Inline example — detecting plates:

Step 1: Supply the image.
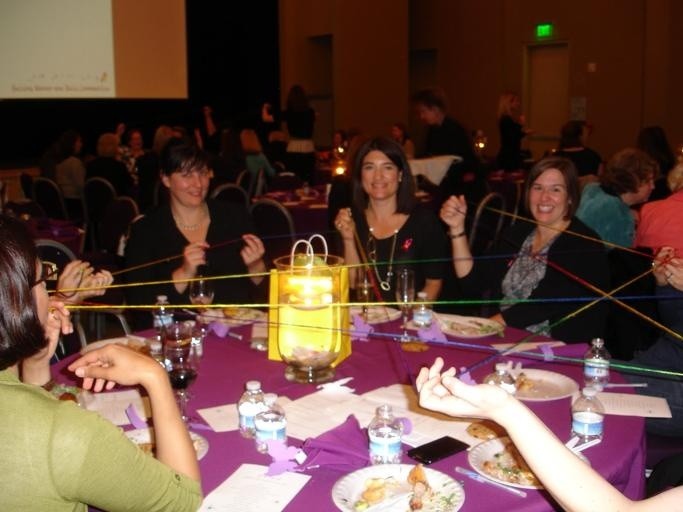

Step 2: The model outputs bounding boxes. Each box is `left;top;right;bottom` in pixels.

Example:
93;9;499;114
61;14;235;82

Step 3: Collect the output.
468;434;591;492
195;309;264;329
432;314;503;342
506;369;581;402
122;426;208;463
330;464;465;512
346;303;401;324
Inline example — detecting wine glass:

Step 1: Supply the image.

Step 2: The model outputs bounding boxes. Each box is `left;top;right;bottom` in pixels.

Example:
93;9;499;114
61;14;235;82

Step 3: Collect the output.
189;275;216;337
354;266;378;327
395;270;417;343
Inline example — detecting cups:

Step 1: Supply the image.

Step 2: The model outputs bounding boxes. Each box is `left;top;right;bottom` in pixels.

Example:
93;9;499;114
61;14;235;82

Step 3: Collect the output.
143;318;205;424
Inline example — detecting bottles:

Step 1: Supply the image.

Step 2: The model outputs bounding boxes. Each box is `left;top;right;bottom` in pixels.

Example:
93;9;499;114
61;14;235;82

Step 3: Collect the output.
239;380;265;437
153;296;173;333
569;384;603;456
583;338;612;384
412;290;433;328
484;363;516;398
367;404;401;465
253;393;286;453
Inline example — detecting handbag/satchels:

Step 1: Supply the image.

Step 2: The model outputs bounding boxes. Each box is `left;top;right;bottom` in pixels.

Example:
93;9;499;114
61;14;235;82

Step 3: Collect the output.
267;234;353;367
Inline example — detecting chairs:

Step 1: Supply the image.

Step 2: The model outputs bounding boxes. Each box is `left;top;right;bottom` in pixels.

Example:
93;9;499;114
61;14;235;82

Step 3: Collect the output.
642;432;683;489
0;127;683;368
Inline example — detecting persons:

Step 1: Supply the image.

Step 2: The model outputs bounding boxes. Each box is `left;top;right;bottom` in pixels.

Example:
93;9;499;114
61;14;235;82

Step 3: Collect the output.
0;85;683;512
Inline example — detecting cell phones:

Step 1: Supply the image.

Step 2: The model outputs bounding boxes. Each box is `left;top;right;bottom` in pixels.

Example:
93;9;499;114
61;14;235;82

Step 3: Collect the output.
406;436;469;465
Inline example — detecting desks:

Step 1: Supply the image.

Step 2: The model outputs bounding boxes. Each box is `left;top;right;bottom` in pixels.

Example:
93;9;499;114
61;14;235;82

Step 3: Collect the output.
44;307;650;512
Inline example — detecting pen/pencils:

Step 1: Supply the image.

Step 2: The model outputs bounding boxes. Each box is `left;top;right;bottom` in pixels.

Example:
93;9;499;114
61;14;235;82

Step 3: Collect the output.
226;332;243;341
455;466;528;498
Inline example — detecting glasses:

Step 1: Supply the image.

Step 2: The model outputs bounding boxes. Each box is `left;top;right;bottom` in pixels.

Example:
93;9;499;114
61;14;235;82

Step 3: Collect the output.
29;261;60;291
